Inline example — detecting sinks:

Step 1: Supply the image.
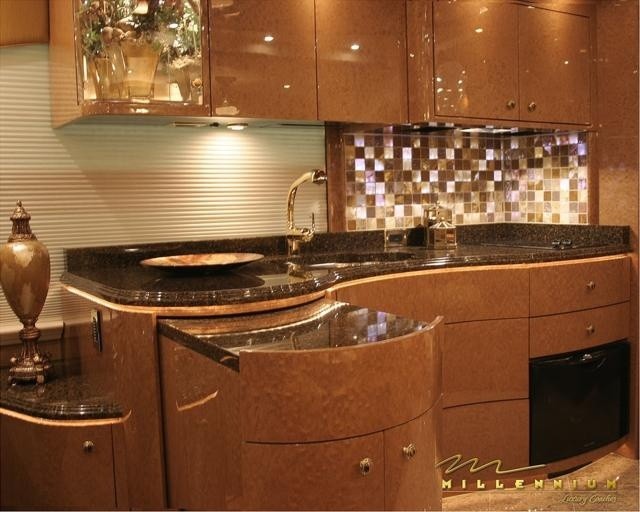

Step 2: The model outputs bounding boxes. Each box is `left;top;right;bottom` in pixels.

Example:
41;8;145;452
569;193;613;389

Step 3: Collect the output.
261;250;415;269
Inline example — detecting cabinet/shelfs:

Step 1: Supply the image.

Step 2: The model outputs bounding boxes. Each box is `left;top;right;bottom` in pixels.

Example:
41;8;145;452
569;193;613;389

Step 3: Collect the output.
435;1;597;131
2;406;128;509
210;1;410;129
160;342;445;511
527;257;630;355
338;268;529;501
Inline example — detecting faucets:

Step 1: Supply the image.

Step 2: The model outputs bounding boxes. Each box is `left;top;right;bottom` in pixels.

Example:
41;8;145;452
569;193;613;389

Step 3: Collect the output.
284;168;330;257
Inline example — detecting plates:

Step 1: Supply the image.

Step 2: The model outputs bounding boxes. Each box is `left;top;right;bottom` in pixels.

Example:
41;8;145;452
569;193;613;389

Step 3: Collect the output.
139;251;265;277
